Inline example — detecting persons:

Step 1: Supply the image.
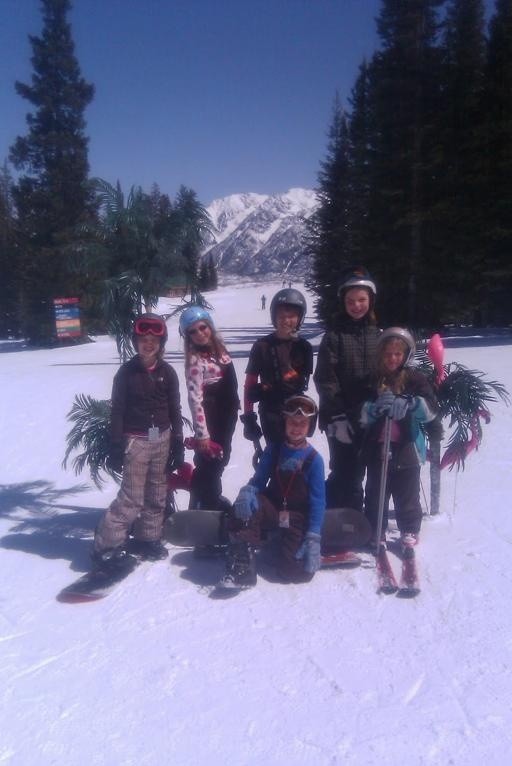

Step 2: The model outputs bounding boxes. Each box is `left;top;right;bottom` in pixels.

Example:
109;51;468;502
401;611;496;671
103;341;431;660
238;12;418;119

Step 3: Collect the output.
176;304;241;515
87;311;188;577
237;285;315;473
339;325;442;598
260;294;267;310
309;273;389;515
212;392;329;595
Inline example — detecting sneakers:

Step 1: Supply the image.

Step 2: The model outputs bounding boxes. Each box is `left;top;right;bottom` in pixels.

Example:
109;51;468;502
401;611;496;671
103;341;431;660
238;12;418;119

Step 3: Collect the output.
90;546;137;573
133;538;170;561
219;565;256;589
193;544;228;560
370;533;388;551
400;532;419;548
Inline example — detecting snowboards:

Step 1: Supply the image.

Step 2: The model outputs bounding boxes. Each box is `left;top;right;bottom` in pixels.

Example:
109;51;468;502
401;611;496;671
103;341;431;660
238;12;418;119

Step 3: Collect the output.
163;507;372;548
64;525;174;600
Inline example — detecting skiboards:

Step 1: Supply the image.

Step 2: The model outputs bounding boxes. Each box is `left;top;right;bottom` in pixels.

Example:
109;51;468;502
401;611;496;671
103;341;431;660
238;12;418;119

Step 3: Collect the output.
369;530;421;595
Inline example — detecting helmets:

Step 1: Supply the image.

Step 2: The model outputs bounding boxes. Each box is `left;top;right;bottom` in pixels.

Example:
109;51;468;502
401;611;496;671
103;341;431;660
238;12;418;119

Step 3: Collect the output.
280;394;318;438
180;306;215;338
336;276;378;296
269;288;307;332
376;327;416;367
131;313;168;353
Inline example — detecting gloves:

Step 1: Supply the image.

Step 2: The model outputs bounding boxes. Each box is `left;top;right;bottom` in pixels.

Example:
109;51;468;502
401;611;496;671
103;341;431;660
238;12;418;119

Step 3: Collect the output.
295;532;321;574
185;437;224;460
233;485;259;521
388;391;416;422
109;443;124;475
246;382;274;404
326;413;356;445
169;434;185;467
240;412;263;441
369;386;396;419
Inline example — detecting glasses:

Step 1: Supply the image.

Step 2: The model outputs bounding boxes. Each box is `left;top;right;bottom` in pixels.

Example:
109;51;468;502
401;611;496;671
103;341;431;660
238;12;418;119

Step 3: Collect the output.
340;267;372;283
135;319;166;338
186;323;208;335
282;397;317;418
